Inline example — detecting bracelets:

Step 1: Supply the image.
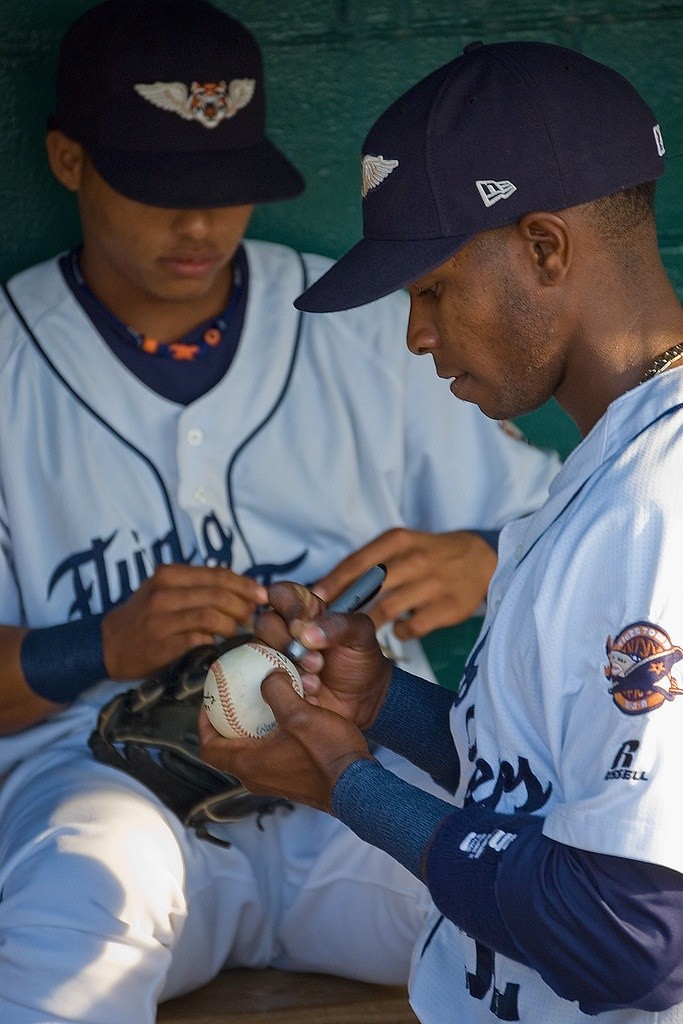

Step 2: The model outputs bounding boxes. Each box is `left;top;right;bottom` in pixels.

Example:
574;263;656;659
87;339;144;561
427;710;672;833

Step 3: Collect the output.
20;613;112;710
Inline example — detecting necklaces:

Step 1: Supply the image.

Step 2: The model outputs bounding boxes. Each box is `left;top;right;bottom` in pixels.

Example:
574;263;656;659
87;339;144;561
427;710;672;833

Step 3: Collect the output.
637;342;683;385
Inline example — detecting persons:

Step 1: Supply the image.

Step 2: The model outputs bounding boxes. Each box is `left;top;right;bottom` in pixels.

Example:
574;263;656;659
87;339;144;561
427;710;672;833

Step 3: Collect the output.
0;3;561;1024
205;40;683;1024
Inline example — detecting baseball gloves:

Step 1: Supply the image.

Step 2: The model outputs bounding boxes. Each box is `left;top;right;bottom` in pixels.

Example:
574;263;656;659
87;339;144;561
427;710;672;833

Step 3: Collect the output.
89;634;296;850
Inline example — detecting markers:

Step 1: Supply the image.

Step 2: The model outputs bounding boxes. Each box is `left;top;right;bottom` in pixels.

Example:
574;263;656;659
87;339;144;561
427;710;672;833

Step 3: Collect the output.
286;563;388;666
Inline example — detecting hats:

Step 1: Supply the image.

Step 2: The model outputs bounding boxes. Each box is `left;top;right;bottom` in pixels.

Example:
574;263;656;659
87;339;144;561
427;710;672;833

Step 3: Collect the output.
293;39;665;314
45;0;305;208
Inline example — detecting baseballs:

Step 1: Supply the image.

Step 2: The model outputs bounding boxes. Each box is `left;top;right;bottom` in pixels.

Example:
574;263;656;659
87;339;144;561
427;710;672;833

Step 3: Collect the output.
204;643;305;740
